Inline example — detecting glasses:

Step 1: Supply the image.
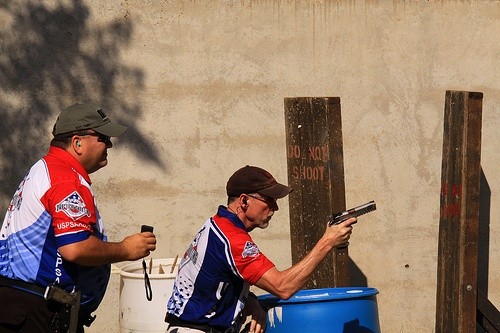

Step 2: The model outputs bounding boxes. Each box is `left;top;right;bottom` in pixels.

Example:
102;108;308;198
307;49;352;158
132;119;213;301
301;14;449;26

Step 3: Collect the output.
65;132;110;143
237;193;277;208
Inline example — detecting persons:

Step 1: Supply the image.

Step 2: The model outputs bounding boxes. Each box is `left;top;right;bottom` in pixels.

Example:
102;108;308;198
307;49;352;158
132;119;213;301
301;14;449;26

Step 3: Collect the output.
164;165;357;333
0;103;157;333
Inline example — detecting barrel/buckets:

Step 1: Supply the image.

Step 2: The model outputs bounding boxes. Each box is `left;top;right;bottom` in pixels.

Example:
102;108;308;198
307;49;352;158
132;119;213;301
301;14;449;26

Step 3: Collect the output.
119;258;183;333
257;287;381;333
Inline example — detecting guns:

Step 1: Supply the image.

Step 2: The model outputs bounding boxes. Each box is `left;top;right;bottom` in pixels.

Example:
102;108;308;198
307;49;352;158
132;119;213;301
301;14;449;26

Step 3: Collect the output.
329;200;377;248
44;284;81;333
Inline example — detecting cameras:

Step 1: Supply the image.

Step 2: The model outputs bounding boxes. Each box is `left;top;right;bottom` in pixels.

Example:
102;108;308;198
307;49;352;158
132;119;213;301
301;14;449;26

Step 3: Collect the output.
141;225;153;233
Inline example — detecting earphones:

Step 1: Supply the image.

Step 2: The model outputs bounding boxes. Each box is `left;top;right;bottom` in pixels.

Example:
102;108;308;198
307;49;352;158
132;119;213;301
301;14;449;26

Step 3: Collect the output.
243;197;247;205
77;141;80;147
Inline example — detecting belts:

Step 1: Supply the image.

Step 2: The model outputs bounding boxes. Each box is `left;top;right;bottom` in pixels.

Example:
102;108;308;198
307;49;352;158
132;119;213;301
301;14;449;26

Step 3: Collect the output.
170;318;226;333
0;275;46;298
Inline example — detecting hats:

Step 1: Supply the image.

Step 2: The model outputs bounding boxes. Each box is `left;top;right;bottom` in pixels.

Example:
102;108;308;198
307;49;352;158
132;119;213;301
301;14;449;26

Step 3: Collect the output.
52;103;128;137
227;165;294;199
